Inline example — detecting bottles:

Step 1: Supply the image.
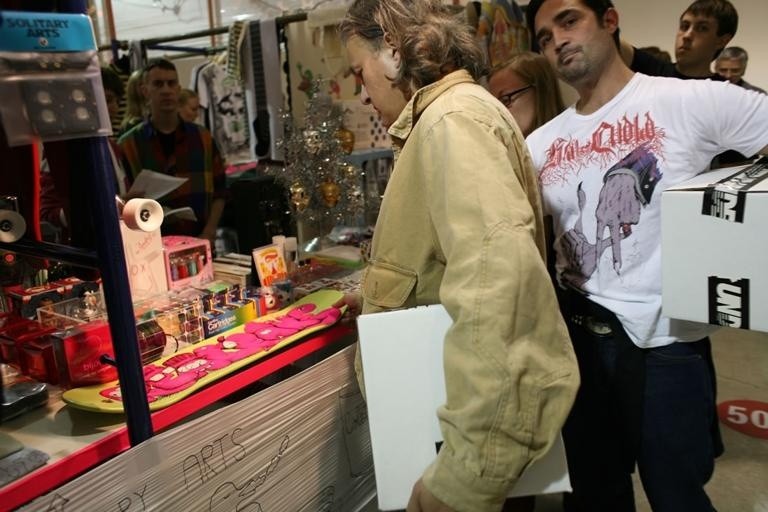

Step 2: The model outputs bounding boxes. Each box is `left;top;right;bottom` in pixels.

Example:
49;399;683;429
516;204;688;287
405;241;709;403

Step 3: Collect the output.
273;236;298;280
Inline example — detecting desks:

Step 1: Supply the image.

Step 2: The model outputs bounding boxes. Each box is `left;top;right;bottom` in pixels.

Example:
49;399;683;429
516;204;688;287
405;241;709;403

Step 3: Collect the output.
0;246;376;512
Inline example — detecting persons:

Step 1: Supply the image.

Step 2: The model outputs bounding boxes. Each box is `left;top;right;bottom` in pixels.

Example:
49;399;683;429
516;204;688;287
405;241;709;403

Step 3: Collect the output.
172;88;200;125
37;65;148;260
711;46;767;170
330;0;589;511
120;58;229;256
510;2;768;512
605;2;765;96
122;68;152;134
483;49;568;280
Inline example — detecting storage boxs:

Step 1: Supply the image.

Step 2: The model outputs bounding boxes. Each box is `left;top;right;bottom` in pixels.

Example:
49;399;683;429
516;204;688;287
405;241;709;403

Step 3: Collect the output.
659;162;768;332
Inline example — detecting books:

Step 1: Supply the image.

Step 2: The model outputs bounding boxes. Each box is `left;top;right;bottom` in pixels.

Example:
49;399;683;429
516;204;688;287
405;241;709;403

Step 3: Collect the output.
126;166;190;208
152;205;197;222
355;298;574;508
